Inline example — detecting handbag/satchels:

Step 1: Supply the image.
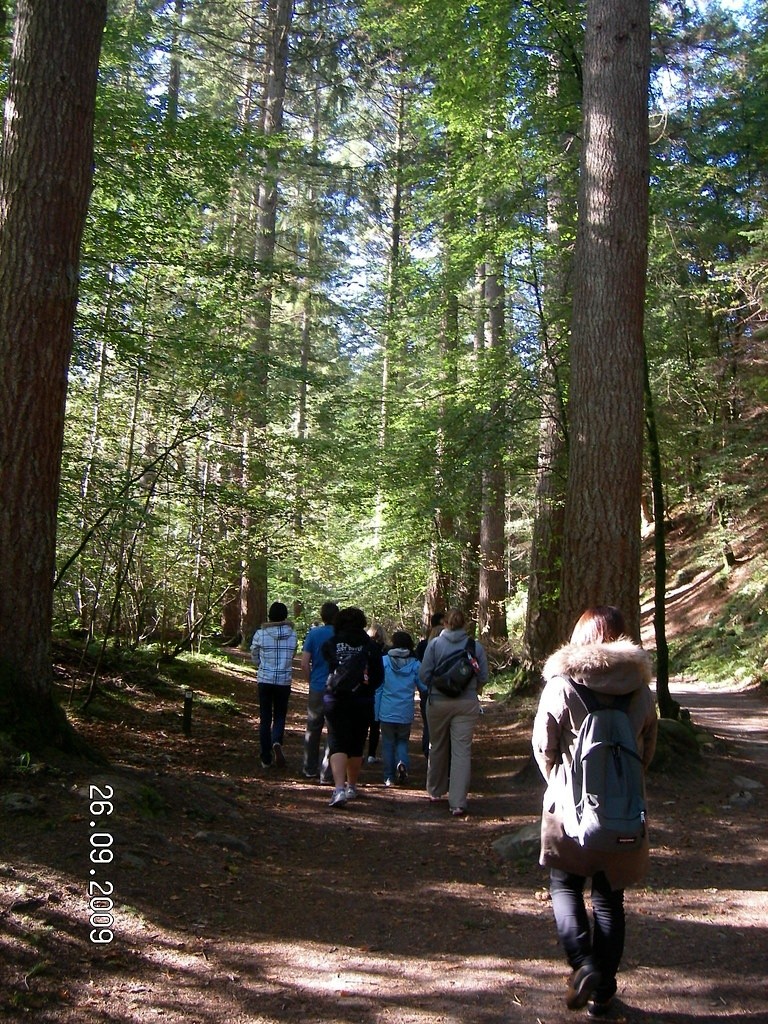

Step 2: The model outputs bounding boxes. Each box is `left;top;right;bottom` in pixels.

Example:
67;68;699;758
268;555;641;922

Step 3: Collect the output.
431;635;479;699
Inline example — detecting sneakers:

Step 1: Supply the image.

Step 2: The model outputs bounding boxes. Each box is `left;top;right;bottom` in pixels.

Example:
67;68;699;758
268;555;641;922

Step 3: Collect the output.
328;786;348;806
344;783;359;799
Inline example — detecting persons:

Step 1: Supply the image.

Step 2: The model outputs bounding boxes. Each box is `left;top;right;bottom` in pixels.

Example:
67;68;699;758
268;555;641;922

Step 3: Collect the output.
533;607;659;1024
358;624;392;761
375;630;429;787
320;606;385;807
414;615;466;780
418;609;491;815
250;602;299;768
303;602;347;784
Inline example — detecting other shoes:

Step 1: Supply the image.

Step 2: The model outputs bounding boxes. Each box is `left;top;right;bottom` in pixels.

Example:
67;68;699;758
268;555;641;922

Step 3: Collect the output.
367;756;383;763
301;766;317;778
587;978;617;1018
449;806;466;815
320;771;333;785
272;742;286;768
396;761;409;780
428;793;441;802
261;759;272;768
566;964;601;1011
384;777;396;787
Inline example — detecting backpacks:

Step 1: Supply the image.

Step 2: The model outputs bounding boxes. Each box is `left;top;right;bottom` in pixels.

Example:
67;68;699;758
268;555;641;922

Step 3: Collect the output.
330;636;372;700
548;680;648;854
